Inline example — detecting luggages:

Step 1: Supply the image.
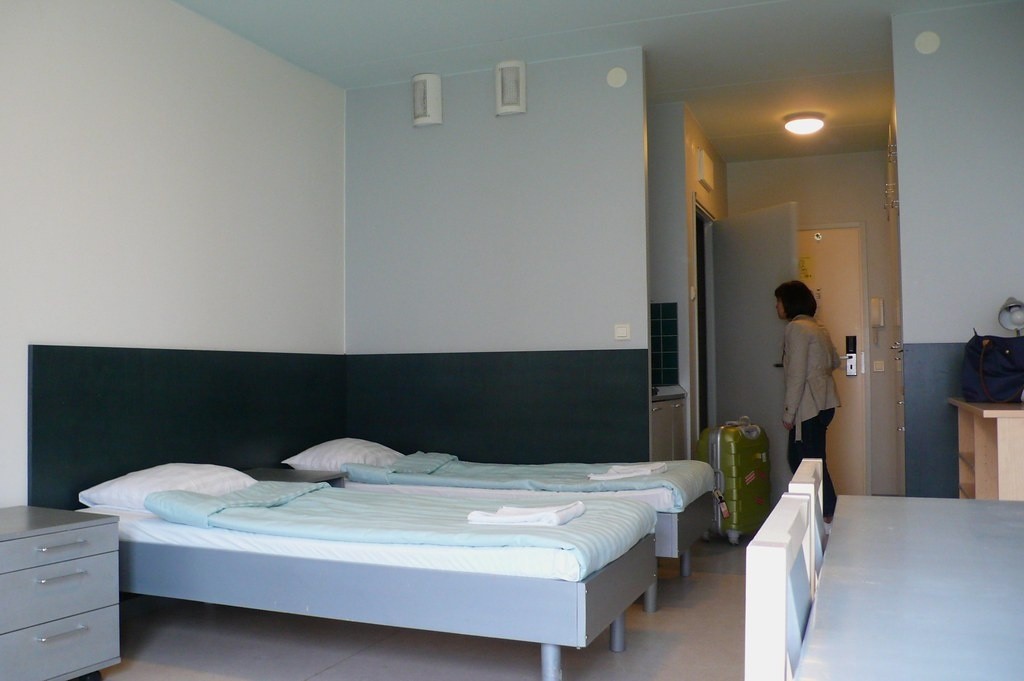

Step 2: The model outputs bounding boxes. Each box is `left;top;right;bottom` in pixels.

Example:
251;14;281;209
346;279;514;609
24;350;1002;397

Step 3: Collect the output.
696;415;770;545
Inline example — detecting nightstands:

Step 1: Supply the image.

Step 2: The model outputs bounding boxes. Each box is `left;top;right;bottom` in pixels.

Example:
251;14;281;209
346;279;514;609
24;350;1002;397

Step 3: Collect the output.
0;505;122;681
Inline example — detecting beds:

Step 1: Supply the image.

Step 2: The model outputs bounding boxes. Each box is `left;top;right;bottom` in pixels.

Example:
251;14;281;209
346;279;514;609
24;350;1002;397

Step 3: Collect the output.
75;437;717;680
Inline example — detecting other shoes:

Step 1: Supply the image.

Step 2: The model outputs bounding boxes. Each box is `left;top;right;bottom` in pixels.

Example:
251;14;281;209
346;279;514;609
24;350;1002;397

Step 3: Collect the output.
824;520;832;535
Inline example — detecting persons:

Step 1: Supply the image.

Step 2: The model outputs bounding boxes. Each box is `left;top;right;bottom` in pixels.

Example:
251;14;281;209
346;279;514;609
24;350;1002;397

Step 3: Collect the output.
775;279;843;535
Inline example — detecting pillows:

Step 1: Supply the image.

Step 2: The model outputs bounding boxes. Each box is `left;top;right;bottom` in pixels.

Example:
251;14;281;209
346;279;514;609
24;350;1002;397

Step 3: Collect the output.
280;438;404;470
77;463;256;514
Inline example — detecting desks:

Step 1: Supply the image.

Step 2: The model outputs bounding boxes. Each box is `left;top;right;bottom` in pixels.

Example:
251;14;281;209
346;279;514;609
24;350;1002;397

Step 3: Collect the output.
795;495;1024;681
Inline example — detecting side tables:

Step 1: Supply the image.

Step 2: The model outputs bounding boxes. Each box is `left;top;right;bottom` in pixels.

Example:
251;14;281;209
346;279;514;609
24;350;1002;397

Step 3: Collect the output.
247;468;348;488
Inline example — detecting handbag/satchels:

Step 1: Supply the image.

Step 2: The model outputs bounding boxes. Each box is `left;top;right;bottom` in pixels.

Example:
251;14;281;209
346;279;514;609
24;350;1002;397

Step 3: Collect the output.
963;328;1024;404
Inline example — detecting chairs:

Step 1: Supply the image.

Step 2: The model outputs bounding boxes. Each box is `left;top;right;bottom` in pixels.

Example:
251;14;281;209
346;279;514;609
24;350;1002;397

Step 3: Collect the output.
743;456;827;681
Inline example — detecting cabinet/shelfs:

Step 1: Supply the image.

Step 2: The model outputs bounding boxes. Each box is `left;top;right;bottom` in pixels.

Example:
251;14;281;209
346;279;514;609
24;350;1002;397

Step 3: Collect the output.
949;396;1024;500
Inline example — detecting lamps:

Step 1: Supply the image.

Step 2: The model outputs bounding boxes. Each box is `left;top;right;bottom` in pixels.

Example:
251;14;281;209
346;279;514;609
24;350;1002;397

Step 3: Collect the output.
998;297;1024;338
494;61;526;116
783;115;823;136
412;74;444;126
